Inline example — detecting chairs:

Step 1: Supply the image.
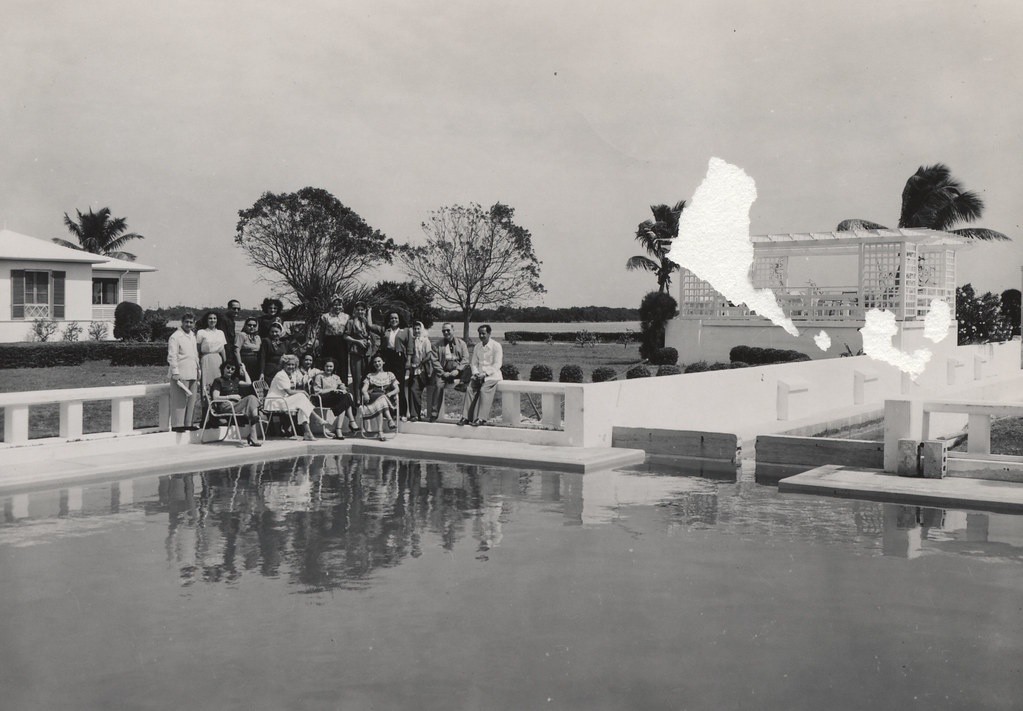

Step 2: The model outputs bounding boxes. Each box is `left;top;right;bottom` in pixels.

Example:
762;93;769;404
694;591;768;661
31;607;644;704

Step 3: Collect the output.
252;380;298;439
200;383;265;447
308;378;354;439
360;393;400;439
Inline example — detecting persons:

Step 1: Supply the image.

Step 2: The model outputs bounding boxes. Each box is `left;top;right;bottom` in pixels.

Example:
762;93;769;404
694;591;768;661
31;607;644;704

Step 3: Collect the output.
360;353;399;442
311;358;362;439
264;353;330;441
210;359;263;446
293;354;325;396
162;291;506;439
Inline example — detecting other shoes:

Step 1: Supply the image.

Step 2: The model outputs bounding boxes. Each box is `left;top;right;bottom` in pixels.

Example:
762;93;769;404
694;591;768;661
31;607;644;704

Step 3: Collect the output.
310;418;328;425
172;427;186;432
303;431;317;441
410;416;421;421
454;384;468;392
429;416;436;422
199;420;220;430
185;426;198;430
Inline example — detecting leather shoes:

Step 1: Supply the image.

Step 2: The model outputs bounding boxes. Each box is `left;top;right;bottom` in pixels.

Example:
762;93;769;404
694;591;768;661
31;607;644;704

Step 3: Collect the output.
470;418;484;425
456;418;469;426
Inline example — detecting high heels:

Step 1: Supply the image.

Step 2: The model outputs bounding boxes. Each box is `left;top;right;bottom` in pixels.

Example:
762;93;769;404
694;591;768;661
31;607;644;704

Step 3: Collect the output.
388;419;397;430
247;433;261;446
250;415;260;427
349;420;360;432
378;431;386;440
334;428;345;440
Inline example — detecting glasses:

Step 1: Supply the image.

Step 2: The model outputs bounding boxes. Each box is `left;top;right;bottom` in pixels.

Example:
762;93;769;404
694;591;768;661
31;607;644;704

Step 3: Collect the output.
230;306;241;311
442;329;450;333
226;366;235;371
247;324;257;327
